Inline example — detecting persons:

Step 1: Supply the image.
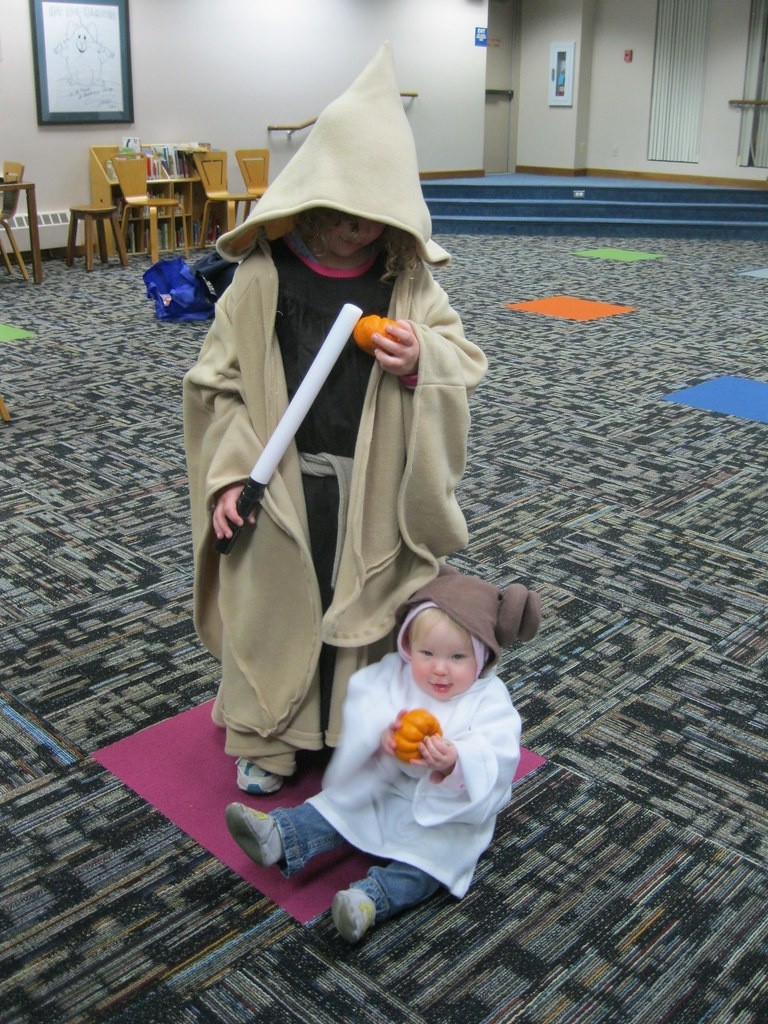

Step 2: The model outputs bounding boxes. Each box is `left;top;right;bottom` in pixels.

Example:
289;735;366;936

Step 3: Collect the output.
227;564;522;945
176;101;489;792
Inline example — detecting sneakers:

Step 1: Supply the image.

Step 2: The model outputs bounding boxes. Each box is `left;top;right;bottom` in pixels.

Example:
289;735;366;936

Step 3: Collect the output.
235;755;283;795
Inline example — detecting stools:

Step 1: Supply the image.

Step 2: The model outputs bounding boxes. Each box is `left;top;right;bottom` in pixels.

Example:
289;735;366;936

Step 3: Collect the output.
65;204;130;275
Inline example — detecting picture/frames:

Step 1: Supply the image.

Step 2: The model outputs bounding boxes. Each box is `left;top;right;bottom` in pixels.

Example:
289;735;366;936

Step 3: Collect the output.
27;1;137;126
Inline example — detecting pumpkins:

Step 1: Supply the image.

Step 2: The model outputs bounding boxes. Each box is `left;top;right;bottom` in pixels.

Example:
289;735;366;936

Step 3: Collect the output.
355;314;404;359
394;709;442;763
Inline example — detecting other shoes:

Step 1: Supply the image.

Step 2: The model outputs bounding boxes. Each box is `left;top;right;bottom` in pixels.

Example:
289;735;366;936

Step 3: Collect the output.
332;887;375;946
225;802;284;869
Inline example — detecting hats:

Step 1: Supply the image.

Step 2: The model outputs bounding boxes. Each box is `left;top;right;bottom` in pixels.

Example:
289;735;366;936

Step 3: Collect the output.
397;600;491;679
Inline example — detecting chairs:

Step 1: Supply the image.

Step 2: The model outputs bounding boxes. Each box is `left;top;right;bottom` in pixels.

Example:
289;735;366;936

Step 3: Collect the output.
110;152;192;264
0;160;30;283
192;152;255;249
237;148;275;224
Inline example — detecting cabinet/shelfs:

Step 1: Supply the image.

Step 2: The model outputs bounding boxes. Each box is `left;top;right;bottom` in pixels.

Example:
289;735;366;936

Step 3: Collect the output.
87;139;230;247
